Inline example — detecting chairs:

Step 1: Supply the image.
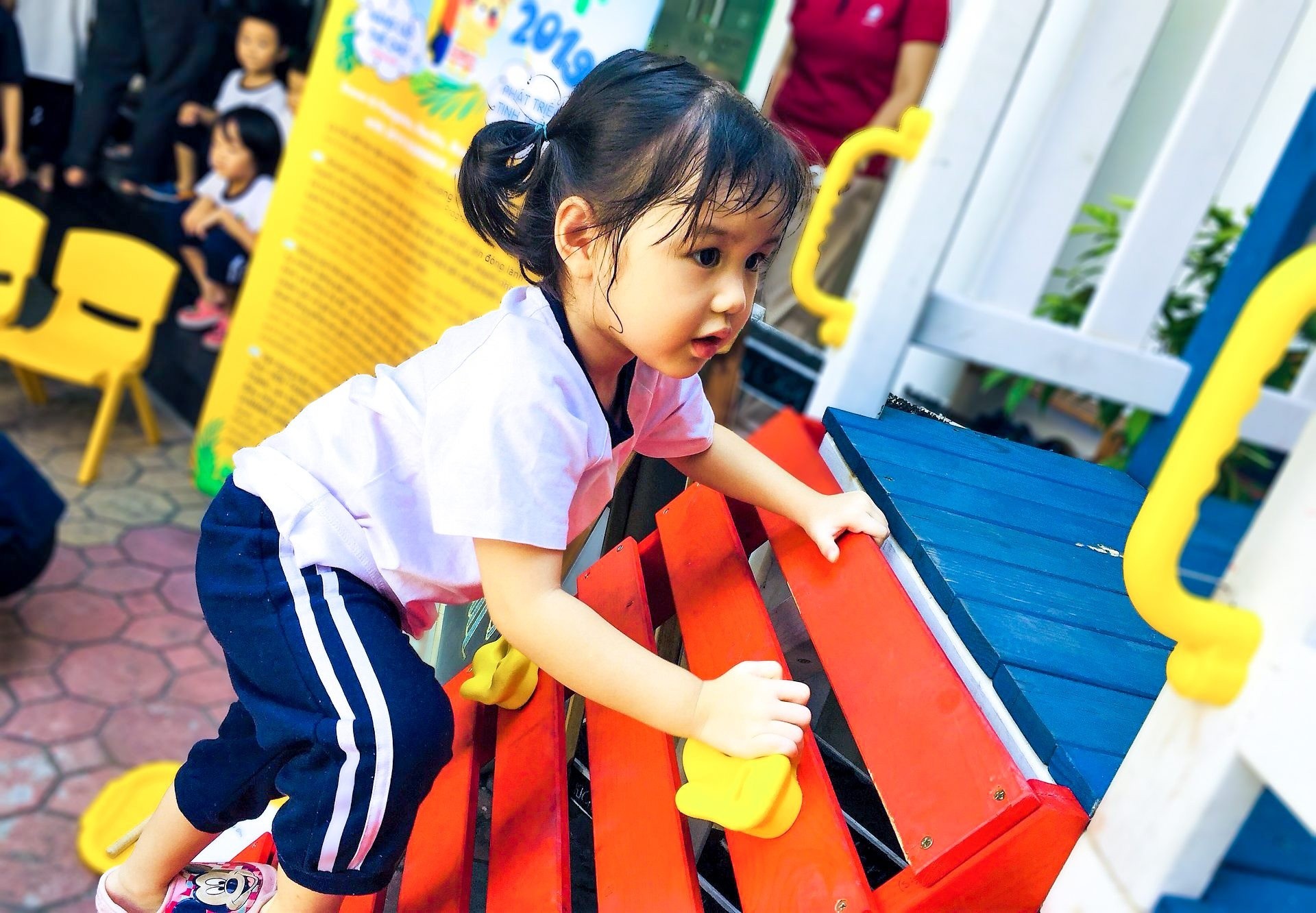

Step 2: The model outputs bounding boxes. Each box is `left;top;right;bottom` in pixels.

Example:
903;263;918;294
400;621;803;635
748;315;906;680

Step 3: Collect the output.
0;190;49;403
0;227;180;489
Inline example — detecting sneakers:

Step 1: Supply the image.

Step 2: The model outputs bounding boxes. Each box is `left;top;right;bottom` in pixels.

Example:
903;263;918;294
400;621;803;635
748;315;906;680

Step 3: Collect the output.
95;861;277;913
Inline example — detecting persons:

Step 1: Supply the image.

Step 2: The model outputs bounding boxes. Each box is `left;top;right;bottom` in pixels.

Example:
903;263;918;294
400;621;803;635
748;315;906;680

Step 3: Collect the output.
177;106;284;351
704;0;952;445
92;49;893;913
0;1;314;205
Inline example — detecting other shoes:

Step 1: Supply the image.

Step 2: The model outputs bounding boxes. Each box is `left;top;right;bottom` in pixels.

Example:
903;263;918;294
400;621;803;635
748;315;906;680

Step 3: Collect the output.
203;314;230;350
177;294;231;327
